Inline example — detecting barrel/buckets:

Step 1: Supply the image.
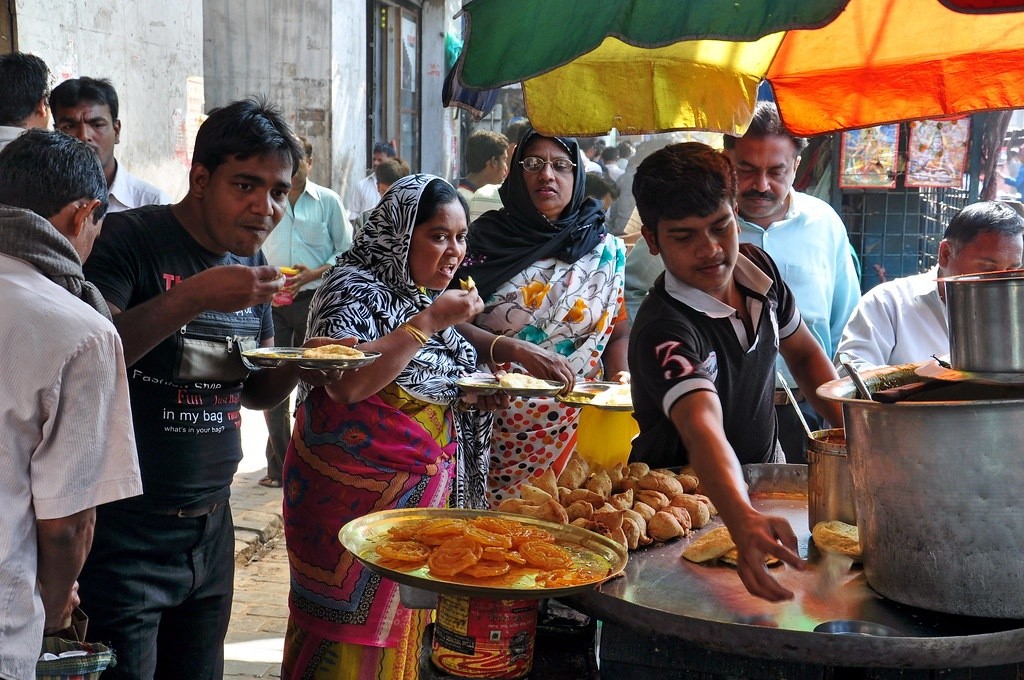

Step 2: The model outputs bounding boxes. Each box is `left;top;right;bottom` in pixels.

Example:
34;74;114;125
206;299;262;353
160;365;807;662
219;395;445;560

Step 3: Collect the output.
803;430;860;537
430;587;537;679
932;269;1024;372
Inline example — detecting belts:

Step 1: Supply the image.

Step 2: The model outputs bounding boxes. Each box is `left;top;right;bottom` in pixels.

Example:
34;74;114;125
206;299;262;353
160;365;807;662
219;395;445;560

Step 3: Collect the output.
152;504;218;518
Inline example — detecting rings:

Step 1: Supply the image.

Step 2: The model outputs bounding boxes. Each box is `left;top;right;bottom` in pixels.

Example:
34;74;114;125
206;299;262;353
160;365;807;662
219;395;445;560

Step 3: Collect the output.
488;409;497;413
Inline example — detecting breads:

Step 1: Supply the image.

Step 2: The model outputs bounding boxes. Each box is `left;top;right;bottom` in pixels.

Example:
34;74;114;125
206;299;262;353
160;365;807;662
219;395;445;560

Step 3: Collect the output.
498;451;718;549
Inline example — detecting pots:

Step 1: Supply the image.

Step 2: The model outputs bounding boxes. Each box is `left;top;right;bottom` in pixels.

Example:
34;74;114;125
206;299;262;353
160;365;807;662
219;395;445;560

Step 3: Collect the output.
815;361;1024;622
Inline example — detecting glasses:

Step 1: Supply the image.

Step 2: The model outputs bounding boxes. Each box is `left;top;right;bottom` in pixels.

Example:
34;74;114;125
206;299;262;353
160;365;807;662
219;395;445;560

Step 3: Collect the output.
519;157;576;174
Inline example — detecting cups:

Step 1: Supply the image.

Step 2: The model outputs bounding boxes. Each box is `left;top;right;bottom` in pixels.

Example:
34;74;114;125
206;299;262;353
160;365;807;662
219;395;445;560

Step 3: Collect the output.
274;267;300;305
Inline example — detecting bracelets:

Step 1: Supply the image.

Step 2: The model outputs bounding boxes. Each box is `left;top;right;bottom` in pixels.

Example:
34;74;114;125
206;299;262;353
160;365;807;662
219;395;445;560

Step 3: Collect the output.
490;335;506;367
400;323;431;348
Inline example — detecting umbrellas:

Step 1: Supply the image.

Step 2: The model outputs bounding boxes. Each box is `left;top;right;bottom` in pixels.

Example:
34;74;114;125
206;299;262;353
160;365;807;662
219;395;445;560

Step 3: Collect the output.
457;3;1018;185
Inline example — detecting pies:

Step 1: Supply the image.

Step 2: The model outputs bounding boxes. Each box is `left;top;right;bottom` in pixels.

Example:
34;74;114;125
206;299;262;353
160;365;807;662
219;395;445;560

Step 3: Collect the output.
459;275;475;292
813;520;861;556
303;344;365;360
501;372;553;389
683;526;780;564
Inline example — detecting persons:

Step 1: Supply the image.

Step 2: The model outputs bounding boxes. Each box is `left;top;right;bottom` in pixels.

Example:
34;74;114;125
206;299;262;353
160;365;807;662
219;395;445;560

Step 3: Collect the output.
446;122;631;511
356;117;727;238
995;130;1024;200
623;142;841;605
833;202;1024;382
281;174;510;680
258;135;354;489
0;128;144;680
0;52;162;213
83;92;358;680
621;101;861;463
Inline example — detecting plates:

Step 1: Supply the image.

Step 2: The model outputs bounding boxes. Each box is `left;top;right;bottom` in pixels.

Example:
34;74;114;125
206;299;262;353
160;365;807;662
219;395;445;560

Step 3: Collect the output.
555;378;633;413
338;509;629;600
240;346;382;369
455;375;564;397
813;619;905;637
